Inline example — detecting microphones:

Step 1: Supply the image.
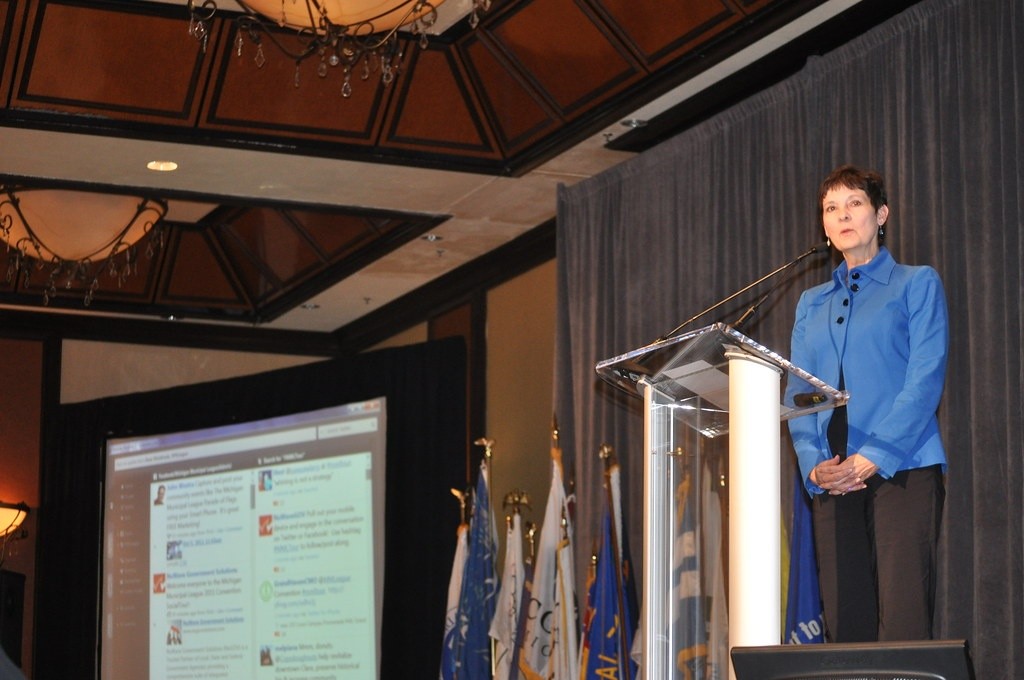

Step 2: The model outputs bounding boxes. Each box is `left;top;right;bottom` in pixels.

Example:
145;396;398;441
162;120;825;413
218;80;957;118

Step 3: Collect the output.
629;232;827;365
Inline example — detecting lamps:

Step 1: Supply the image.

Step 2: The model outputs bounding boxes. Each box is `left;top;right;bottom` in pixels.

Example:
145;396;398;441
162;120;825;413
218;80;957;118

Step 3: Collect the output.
187;0;492;96
0;501;32;564
0;185;219;303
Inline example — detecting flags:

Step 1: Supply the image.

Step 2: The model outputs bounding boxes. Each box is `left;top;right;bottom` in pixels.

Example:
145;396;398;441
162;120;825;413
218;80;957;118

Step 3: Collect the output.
435;412;824;680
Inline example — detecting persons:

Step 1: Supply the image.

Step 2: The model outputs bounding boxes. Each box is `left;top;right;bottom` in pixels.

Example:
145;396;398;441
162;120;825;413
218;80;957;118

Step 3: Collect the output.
258;471;271;491
783;164;951;641
154;486;165;503
